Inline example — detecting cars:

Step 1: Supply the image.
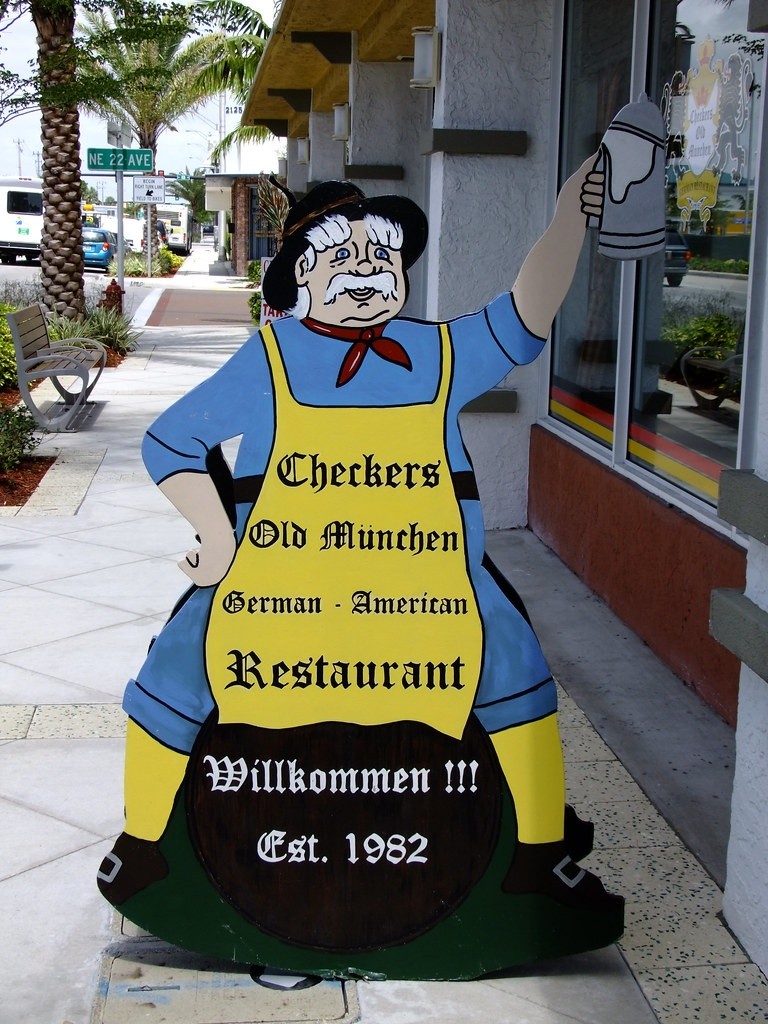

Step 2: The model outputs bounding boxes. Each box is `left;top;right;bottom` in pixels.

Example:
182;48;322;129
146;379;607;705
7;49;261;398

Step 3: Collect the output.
112;232;134;254
80;226;118;273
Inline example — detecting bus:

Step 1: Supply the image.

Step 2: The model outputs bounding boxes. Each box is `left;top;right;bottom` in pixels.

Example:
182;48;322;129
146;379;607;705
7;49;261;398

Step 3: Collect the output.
156;195;198;256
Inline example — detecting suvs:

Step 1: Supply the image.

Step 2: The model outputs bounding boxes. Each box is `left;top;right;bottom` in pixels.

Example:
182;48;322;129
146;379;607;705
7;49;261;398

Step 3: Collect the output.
666;227;692;288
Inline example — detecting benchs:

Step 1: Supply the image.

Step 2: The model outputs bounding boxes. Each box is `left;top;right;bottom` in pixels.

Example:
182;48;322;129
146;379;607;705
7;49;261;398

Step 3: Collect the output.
680;321;744;410
6;303;107;433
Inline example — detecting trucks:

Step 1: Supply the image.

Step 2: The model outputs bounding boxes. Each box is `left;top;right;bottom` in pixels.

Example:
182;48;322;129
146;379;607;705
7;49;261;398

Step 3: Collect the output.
0;177;44;264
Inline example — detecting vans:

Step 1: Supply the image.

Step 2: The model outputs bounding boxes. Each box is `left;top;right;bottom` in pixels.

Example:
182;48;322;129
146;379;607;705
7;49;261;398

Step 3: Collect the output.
156;220;167;245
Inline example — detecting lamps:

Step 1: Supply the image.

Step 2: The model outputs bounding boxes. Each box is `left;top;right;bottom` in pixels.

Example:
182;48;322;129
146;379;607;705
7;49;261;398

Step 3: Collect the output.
331;101;352;142
295;137;310;165
277;157;287;180
409;26;441;90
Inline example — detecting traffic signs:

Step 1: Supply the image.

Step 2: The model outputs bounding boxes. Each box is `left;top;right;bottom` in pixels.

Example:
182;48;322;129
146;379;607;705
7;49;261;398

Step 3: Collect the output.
133;176;165;205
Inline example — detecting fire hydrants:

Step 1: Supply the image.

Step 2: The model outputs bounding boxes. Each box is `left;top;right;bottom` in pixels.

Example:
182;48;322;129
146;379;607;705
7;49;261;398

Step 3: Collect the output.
97;278;125;317
